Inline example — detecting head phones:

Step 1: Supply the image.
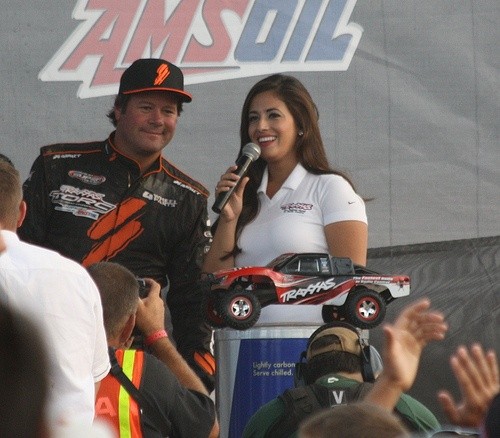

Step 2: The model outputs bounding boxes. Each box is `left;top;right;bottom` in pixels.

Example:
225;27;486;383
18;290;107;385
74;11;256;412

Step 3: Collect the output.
294;321;384;390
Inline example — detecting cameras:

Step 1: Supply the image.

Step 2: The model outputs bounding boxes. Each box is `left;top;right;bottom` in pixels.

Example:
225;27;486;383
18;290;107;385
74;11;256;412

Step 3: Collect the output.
137;279;148;299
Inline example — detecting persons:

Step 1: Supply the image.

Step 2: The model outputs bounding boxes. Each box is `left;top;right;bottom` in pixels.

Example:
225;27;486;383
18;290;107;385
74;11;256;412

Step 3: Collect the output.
242;296;500;438
201;73;368;281
0;58;218;438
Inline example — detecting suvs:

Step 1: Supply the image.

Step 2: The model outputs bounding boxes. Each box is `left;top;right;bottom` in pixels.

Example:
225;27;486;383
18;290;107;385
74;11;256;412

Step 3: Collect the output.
204;252;413;330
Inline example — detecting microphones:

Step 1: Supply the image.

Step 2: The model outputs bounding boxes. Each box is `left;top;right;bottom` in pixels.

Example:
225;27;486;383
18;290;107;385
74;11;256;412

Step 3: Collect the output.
211;143;261;214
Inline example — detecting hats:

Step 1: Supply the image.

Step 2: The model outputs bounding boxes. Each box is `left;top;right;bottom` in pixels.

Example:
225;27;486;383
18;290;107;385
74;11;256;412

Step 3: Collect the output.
306;326;366;361
118;58;193;104
